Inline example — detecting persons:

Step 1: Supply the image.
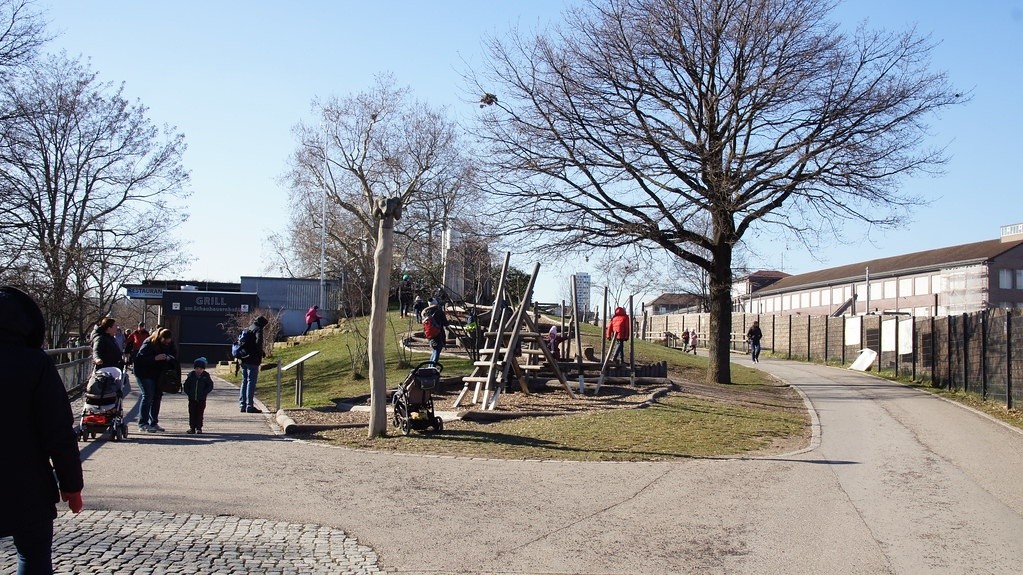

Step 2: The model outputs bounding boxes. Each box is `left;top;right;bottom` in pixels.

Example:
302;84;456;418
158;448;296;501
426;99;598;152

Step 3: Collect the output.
114;321;163;373
89;317;124;374
183;357;214;434
398;274;413;319
301;305;324;336
133;327;178;433
238;315;269;413
0;284;84;575
686;329;697;356
606;306;629;364
545;326;558;362
413;287;450;367
681;327;690;351
747;321;763;364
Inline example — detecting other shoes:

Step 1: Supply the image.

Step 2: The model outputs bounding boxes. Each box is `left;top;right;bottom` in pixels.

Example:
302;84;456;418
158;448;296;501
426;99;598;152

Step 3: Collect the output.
620;358;624;363
187;429;196;433
151;425;165;431
612;356;620;362
241;408;246;412
139;424;157;433
197;429;202;433
246;407;262;413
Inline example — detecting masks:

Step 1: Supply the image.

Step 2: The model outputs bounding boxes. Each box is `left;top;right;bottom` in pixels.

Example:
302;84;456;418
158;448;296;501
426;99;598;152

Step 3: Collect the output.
316;309;318;311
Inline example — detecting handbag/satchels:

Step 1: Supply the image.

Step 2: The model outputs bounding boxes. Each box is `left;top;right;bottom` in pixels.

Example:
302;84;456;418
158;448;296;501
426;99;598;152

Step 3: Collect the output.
159;355;182;394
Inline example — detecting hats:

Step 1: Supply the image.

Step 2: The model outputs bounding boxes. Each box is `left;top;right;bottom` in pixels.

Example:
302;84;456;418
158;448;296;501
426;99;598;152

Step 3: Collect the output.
138;321;145;327
194;357;207;369
549;326;557;343
314;305;318;309
416;296;421;301
403;275;409;279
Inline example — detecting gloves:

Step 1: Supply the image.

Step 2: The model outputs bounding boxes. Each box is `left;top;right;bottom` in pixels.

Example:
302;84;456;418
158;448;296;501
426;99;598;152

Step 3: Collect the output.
61;491;83;513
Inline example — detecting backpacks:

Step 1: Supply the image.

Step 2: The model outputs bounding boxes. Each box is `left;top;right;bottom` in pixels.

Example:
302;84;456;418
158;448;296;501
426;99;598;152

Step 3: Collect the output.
232;327;261;359
422;311;442;339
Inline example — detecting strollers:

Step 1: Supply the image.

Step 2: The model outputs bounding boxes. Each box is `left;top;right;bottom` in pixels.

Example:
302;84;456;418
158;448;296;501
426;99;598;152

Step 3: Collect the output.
79;357;128;443
391;360;444;437
